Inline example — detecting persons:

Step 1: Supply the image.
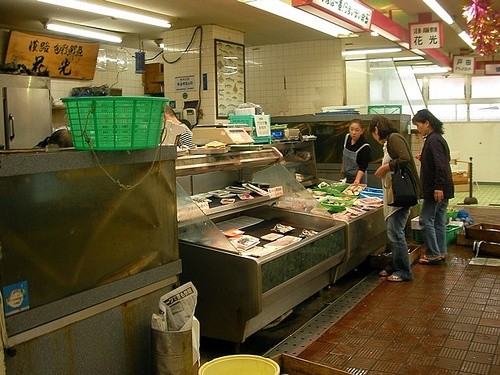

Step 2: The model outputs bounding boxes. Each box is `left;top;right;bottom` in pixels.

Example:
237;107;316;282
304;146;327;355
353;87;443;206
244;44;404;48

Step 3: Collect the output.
341;119;371;186
368;116;421;281
164;105;195;149
411;109;456;265
36;127;73;150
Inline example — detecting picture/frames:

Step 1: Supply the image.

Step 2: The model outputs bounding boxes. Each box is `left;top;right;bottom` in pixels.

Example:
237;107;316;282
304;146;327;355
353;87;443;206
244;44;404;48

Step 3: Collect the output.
214;38;246;119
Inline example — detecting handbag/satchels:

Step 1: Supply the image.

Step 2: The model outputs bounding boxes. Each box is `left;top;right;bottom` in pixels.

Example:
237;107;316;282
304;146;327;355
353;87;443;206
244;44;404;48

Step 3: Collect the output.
383;170;419;208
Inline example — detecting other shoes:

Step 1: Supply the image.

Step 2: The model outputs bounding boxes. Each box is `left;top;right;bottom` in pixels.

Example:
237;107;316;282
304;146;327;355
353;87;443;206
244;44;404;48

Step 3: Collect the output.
386;273;404;282
440;253;447;259
418;254;443;265
378;269;389;276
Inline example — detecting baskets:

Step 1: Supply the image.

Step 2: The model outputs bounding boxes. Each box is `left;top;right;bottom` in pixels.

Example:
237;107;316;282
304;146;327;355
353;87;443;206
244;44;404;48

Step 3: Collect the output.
60;95;171;152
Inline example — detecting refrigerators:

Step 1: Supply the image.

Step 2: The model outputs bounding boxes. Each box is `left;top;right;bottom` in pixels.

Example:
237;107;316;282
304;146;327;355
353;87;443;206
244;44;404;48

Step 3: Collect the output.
0;73;52;150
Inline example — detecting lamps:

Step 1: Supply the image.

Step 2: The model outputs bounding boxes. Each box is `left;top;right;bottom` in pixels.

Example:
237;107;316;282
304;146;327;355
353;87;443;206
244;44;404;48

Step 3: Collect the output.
36;0;172;29
44;18;125;44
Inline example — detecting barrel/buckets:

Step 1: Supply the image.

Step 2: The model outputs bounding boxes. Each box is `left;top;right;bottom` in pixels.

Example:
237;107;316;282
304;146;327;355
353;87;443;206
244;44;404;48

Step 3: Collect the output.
197;354;281;375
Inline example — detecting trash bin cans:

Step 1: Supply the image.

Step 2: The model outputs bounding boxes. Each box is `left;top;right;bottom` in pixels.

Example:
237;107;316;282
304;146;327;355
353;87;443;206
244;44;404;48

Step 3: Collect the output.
150;325;198;375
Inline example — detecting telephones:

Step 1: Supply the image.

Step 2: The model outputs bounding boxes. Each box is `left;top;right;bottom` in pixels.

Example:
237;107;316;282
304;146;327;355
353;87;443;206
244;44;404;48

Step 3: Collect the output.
183;108;196;125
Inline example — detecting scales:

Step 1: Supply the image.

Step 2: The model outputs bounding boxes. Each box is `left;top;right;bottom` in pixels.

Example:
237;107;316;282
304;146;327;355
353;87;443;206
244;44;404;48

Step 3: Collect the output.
191;124;255;147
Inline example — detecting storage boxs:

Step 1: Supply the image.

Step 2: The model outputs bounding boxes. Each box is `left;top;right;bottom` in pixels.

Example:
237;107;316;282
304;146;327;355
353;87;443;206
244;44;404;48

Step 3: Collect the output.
451;171;470;192
371;202;500;271
